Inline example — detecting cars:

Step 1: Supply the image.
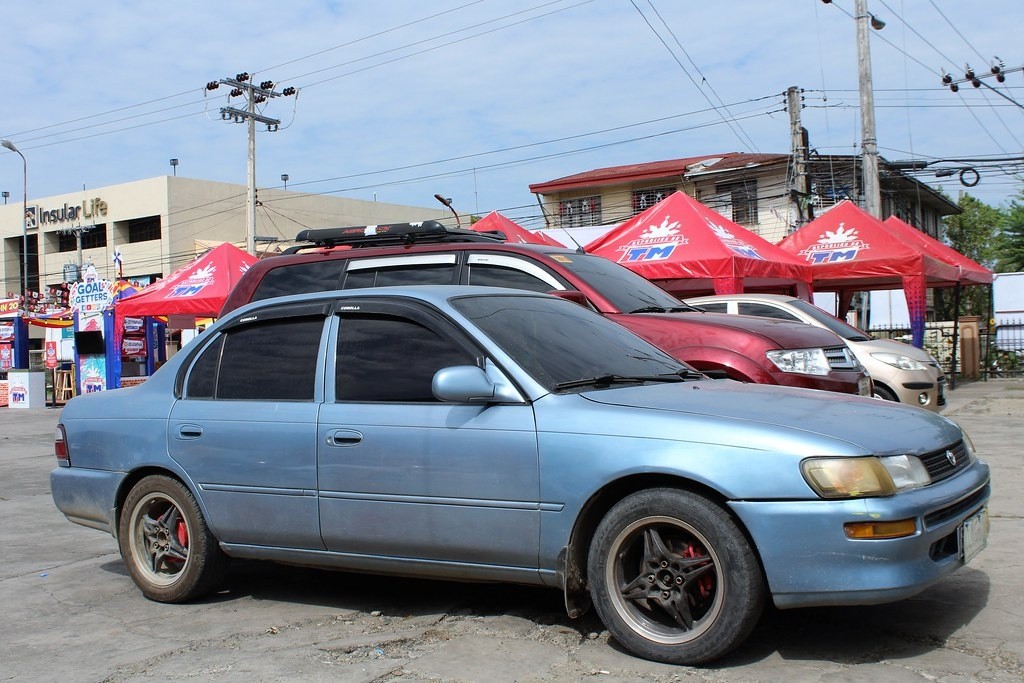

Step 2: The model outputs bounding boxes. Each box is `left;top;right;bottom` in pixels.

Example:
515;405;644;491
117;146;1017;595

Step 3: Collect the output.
674;294;949;412
53;281;994;672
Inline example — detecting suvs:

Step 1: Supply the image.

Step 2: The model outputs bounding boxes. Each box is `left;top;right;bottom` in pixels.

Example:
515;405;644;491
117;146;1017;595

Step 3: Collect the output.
214;224;878;402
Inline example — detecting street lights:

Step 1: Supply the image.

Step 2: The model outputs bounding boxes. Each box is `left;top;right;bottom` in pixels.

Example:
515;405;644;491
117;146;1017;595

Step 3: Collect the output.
0;139;30;317
